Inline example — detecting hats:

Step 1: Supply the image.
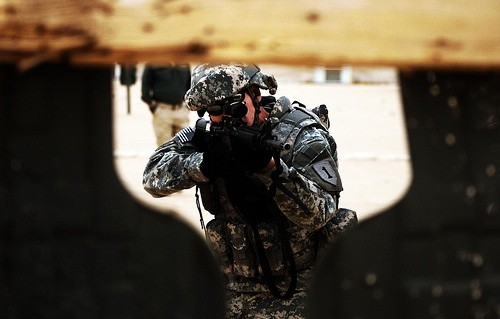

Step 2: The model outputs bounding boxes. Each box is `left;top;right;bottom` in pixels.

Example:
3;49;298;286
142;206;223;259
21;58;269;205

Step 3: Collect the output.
184;62;260;111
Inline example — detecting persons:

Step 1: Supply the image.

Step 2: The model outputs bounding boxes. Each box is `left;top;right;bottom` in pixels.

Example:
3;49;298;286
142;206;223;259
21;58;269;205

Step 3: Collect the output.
140;63;191;148
143;62;358;319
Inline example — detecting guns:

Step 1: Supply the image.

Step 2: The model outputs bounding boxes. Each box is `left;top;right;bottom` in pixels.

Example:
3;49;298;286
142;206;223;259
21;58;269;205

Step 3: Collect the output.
191;92;290;171
